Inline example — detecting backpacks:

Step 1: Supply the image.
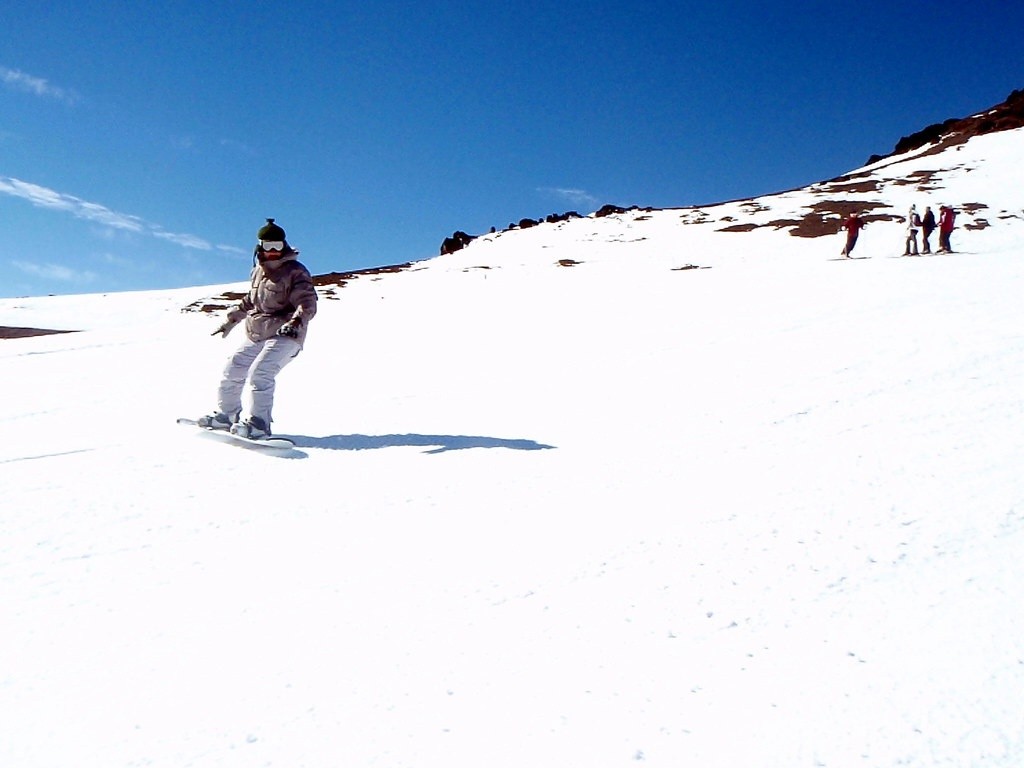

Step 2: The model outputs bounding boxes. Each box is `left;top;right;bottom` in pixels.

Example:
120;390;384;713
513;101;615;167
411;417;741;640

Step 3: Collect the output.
912;213;921;226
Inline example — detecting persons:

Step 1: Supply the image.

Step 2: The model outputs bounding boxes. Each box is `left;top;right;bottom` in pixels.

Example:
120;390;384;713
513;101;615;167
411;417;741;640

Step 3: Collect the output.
920;206;937;254
902;205;922;257
841;211;864;257
199;218;317;439
936;205;954;254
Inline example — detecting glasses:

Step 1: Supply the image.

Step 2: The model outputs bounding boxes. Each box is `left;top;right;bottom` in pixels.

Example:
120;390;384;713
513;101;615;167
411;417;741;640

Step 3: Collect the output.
262;240;284;251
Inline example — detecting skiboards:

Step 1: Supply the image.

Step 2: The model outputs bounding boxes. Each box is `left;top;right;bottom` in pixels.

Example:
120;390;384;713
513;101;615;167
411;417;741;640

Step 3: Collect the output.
888;251;982;258
825;256;873;261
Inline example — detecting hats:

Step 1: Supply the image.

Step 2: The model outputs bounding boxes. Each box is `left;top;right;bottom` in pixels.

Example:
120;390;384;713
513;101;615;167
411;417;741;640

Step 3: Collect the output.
909;204;915;211
257;219;286;252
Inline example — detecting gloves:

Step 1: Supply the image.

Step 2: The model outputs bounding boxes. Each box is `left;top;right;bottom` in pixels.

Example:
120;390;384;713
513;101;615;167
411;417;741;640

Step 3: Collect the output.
276;319;302;339
211;320;238;338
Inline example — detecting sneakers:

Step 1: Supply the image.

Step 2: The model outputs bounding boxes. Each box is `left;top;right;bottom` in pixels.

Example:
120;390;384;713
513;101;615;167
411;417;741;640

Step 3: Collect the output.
230;415;271;439
198;411;241;430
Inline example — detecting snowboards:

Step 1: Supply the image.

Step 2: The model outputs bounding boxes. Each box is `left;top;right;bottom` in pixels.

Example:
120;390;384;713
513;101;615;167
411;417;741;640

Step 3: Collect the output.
176;418;296;450
